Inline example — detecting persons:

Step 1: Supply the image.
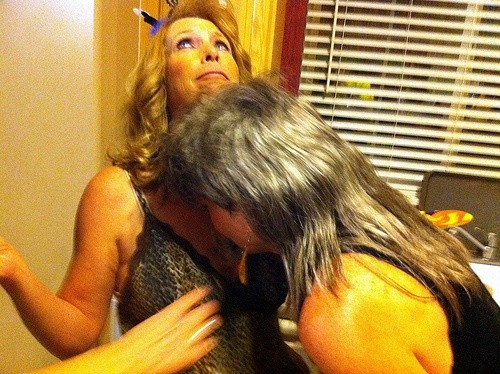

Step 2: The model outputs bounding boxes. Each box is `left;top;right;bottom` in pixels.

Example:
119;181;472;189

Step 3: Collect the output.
157;79;500;374
0;0;313;374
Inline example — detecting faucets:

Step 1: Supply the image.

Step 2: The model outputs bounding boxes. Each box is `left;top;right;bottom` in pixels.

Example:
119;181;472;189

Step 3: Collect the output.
450;222;498;260
